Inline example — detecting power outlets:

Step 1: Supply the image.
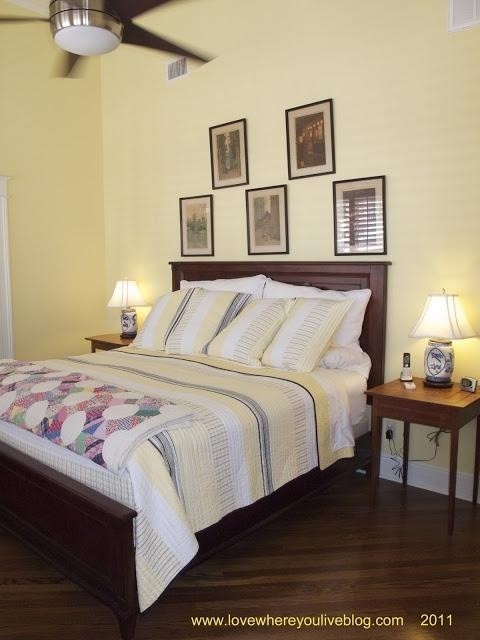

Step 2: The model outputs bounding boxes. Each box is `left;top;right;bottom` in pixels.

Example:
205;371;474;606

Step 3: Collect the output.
382;420;395;439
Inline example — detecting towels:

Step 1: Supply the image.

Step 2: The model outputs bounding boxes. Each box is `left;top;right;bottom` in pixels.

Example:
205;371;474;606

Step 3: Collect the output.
1;359;194;476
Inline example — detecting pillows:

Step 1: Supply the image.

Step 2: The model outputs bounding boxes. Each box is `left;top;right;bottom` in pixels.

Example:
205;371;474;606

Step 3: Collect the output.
207;297;302;369
128;288;201;351
263;278;372;380
261;298;355;373
180;274;267;299
163;288;255;355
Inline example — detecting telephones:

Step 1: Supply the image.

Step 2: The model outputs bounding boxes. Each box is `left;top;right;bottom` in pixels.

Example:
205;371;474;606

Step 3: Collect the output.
400;352;412;382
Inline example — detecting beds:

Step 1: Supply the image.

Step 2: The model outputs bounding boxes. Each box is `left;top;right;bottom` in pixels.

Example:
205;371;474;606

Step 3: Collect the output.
0;262;394;640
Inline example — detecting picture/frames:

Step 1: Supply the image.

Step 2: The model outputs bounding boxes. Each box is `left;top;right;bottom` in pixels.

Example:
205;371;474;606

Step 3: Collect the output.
333;176;387;255
209;118;249;189
179;195;214;256
245;185;289;255
285;99;336;179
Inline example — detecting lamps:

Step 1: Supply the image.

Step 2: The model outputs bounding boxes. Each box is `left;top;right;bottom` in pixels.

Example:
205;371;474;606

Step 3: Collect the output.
107;280;149;339
409;288;479;388
49;10;124;56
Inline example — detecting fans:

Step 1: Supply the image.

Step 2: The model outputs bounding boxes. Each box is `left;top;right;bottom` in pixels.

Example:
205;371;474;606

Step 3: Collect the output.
0;1;207;77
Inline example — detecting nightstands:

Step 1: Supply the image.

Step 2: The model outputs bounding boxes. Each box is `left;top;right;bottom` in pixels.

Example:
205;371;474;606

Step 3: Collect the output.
85;333;134;354
364;378;480;534
